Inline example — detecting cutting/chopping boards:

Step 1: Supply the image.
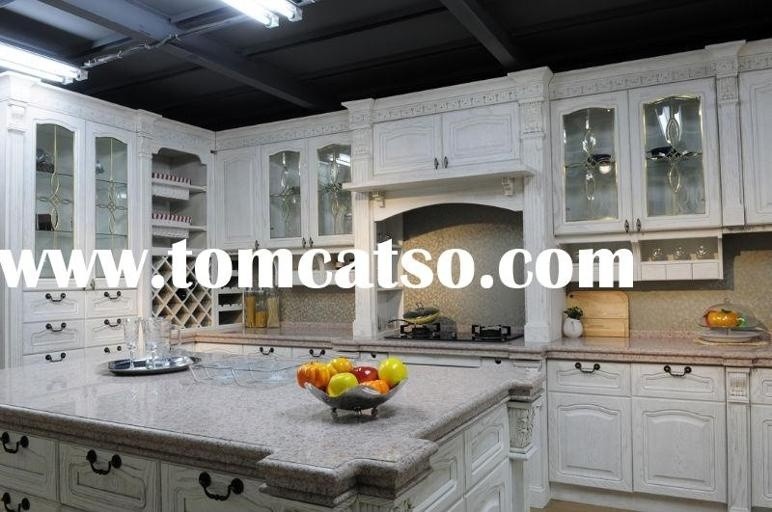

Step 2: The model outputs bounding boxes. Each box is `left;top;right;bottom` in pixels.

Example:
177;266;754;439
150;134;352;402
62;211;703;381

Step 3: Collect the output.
566;291;630;339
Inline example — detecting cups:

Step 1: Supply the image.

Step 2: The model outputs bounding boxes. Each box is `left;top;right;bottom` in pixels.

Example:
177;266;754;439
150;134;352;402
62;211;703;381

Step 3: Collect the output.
140;316;180;369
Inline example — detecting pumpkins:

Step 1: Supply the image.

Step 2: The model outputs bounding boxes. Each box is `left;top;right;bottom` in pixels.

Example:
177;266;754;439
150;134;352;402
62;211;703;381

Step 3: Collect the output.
296;358;353;389
705;308;744;327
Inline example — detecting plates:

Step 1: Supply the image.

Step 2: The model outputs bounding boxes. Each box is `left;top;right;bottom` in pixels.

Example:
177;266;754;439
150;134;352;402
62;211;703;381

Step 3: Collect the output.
696;330;761;344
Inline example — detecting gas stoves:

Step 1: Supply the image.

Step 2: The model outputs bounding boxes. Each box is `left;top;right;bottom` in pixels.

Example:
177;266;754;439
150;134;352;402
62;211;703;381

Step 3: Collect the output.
384;323;524;343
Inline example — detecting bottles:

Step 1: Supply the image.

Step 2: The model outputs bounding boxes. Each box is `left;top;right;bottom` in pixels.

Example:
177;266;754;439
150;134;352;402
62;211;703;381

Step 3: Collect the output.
255;286;266;328
267;287;280;328
245;285;255;328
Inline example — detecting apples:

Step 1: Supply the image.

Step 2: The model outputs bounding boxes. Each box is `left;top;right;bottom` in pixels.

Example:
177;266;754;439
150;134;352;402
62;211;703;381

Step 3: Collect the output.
329;358;408;397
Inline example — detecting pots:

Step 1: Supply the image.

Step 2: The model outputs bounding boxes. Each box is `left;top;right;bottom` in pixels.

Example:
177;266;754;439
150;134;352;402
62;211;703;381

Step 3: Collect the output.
387;302;440;325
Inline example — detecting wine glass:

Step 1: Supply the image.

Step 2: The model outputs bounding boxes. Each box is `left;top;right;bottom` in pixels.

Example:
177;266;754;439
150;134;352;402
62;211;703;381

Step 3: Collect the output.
120;314;142;368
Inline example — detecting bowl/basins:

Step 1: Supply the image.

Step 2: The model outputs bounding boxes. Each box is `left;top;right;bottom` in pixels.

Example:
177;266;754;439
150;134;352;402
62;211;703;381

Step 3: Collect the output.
650;146;676;156
586;154;611;163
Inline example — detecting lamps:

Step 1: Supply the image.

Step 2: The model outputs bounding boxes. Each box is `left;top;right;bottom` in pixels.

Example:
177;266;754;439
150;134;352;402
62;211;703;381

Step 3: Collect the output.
0;42;88;85
224;0;303;28
